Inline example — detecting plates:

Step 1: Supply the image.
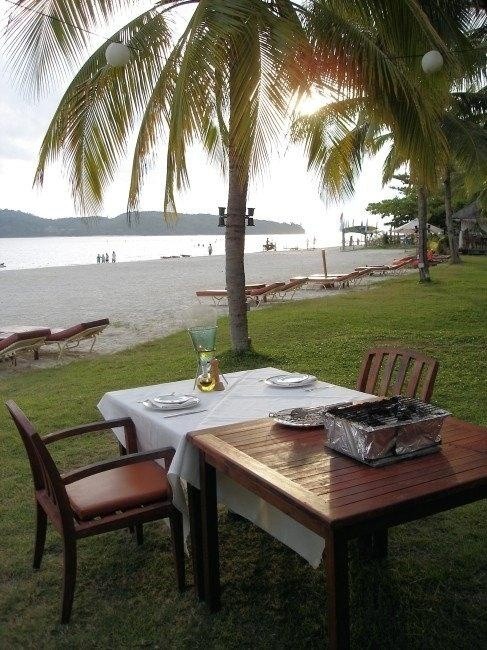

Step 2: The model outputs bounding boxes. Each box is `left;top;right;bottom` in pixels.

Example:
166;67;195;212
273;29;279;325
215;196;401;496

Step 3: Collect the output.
154;394;190;404
273;408;328;427
271;373;309;383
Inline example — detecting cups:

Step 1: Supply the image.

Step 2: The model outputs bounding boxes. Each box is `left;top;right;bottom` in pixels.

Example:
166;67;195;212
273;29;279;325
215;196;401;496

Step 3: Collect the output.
188;326;218;392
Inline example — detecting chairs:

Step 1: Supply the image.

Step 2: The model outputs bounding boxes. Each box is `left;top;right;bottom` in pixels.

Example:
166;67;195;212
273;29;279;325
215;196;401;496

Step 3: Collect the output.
0;329;51;366
1;319;110;359
5;400;186;625
357;348;440;404
195;254;451;310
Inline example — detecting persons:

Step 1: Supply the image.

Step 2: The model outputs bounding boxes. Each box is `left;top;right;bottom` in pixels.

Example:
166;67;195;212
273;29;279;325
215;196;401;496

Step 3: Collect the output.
104;253;109;262
111;251;116;262
207;244;212;255
95;253;100;263
100;254;105;263
